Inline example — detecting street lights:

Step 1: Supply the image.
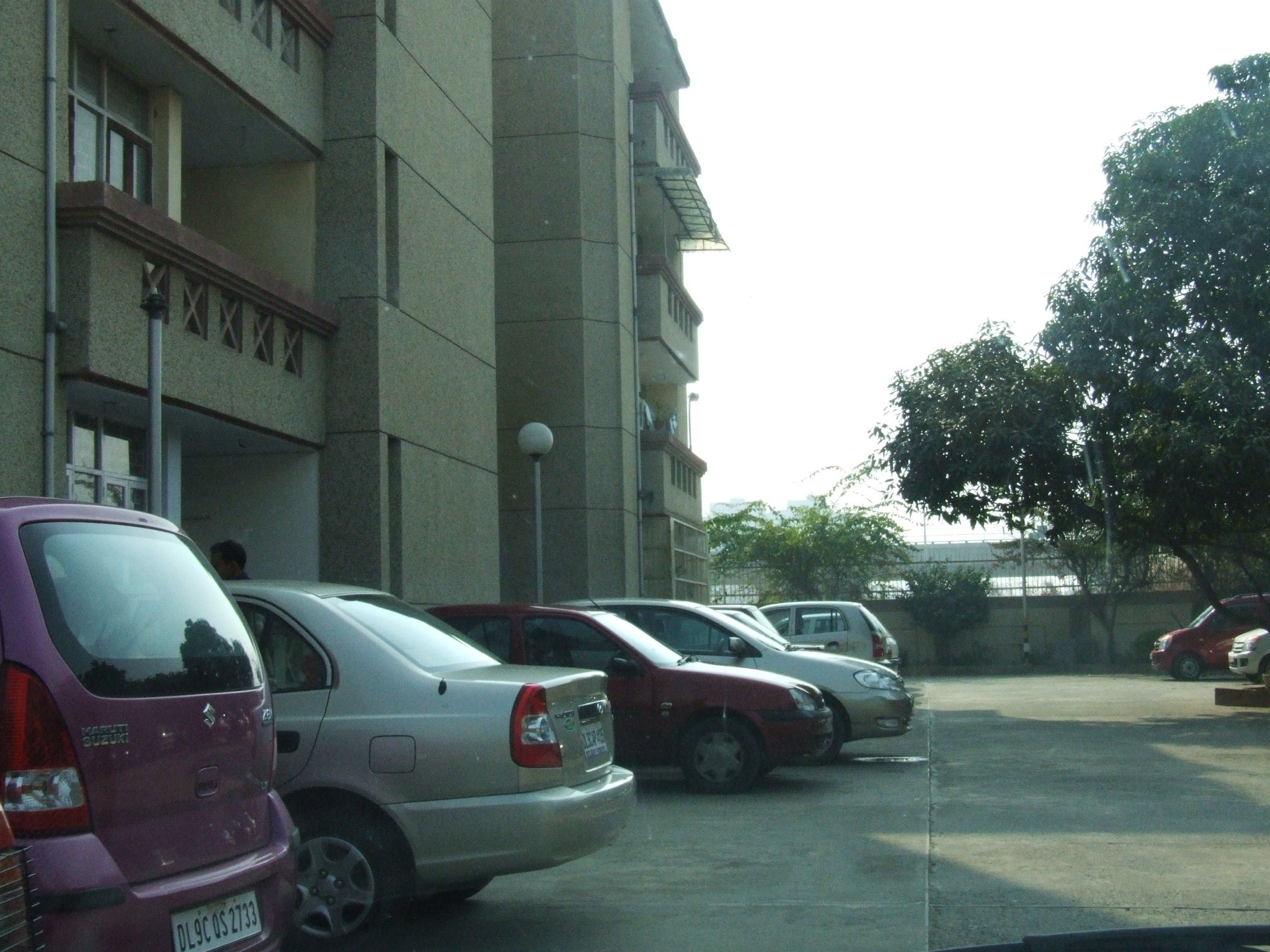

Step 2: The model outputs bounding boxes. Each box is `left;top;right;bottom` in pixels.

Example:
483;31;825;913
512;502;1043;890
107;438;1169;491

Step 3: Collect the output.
518;419;556;601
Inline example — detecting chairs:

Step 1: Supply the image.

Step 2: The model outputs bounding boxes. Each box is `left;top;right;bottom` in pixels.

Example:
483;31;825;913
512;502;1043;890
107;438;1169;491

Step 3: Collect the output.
641;616;664;640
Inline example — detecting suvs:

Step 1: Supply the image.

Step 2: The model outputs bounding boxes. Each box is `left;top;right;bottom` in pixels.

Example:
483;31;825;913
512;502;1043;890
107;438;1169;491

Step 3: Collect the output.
1148;595;1270;681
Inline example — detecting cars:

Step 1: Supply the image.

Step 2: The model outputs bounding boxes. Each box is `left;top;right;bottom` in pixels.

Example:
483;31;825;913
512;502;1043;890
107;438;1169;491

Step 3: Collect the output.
224;584;632;952
428;601;912;793
0;494;300;952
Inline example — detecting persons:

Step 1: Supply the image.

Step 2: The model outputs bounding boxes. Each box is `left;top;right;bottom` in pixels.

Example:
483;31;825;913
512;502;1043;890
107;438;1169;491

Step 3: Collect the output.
210;539;250;580
